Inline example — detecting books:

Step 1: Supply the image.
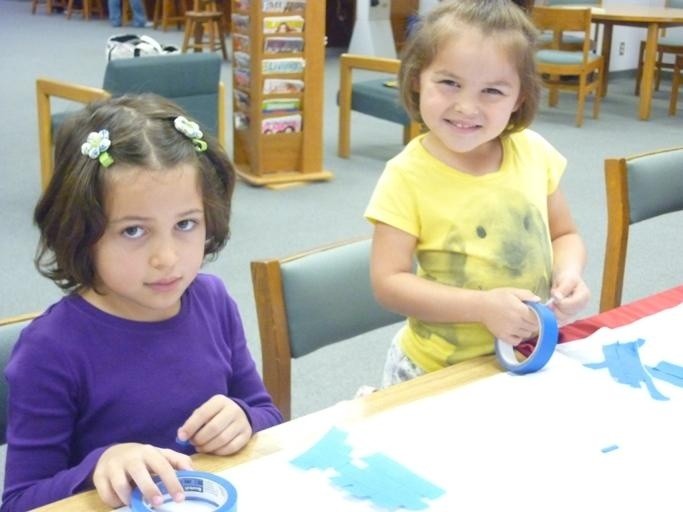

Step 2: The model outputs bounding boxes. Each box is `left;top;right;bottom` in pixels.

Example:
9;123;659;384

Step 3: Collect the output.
230;1;305;136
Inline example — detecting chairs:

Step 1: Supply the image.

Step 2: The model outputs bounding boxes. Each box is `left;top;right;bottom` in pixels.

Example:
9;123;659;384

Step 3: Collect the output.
39;55;227;188
600;149;683;313
339;53;424;159
527;2;681;128
251;237;418;422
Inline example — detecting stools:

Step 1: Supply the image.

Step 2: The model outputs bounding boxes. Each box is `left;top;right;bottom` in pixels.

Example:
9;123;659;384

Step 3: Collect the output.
181;11;228;60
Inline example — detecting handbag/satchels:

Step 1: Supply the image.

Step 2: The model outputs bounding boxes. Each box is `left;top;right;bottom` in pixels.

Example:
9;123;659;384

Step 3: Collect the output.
106;33;181;62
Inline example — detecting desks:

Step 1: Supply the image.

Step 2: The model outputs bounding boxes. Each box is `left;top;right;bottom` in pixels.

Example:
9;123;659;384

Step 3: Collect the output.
22;289;682;512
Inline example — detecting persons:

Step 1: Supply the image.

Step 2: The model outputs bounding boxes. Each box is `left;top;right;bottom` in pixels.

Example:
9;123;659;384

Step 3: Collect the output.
0;92;282;510
346;1;396;85
362;1;590;380
107;1;155;30
